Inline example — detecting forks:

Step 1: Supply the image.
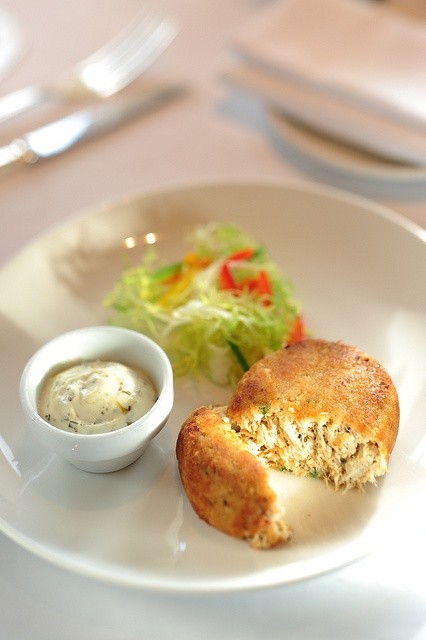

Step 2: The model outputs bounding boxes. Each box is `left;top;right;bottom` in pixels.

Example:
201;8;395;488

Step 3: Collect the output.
0;12;183;125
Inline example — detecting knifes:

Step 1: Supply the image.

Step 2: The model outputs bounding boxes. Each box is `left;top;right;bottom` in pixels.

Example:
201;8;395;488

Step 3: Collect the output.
0;78;183;169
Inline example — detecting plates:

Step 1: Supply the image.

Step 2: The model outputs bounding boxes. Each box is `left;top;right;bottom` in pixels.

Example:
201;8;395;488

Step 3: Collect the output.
267;101;425;201
1;173;426;596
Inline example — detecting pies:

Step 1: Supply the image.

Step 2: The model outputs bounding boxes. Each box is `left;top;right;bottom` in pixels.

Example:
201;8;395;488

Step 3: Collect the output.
176;339;400;550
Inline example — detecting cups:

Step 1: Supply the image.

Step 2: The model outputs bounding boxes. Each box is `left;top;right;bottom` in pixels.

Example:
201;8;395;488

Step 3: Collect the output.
17;324;176;476
